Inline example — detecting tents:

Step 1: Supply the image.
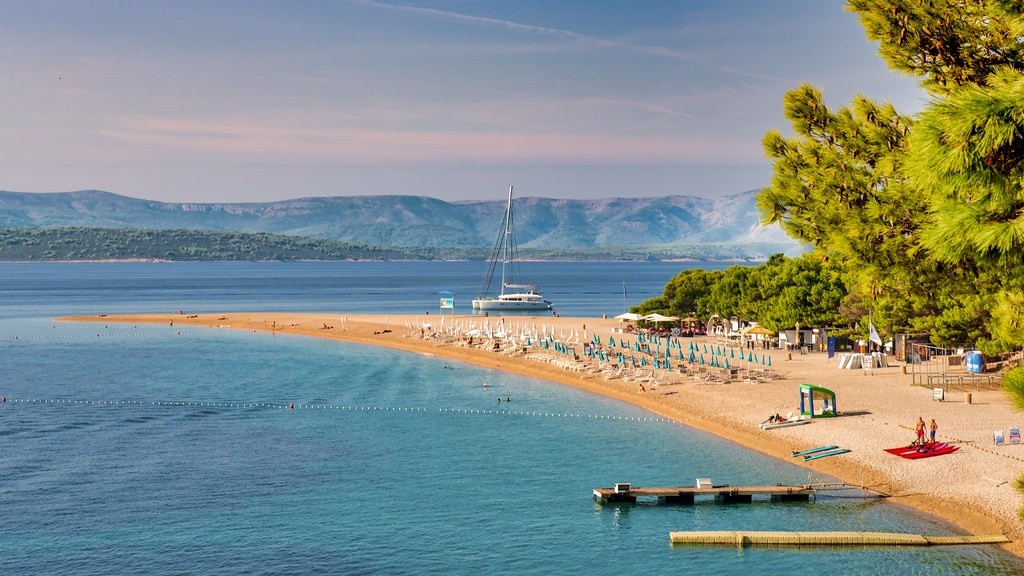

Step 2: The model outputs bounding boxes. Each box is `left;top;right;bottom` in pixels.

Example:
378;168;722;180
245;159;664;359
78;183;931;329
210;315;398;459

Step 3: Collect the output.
745;324;775;350
614;312;679;336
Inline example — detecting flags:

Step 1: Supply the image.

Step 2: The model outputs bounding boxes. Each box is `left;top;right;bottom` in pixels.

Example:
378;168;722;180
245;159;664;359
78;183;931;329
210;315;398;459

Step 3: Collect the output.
870;320;882;346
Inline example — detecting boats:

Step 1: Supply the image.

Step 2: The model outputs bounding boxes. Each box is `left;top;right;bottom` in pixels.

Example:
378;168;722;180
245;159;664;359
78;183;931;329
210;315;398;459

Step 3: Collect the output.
471;185;550;311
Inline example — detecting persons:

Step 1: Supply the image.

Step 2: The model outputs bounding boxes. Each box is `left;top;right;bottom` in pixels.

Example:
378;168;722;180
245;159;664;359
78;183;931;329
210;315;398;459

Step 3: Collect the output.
930;418;938;443
914;416;927;445
